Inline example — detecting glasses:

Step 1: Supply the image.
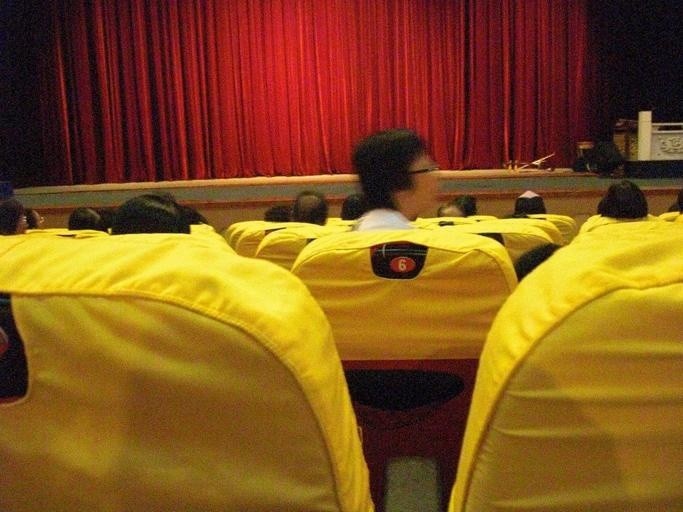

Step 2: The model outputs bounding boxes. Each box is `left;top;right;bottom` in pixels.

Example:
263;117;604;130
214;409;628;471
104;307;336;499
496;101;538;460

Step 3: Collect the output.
407;165;441;178
21;215;45;225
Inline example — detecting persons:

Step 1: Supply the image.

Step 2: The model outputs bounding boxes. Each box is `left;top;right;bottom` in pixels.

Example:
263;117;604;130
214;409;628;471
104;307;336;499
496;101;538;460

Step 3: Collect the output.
353;128;444;231
1;193;209;235
437;180;683;219
264;190;371;226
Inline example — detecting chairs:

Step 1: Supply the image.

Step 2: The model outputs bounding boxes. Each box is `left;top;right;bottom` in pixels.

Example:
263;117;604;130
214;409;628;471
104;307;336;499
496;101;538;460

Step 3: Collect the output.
0;211;683;512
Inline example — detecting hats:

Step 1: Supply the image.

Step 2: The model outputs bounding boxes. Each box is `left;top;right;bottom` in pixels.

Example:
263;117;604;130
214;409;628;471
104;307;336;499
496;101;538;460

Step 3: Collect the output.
514;191;545;217
